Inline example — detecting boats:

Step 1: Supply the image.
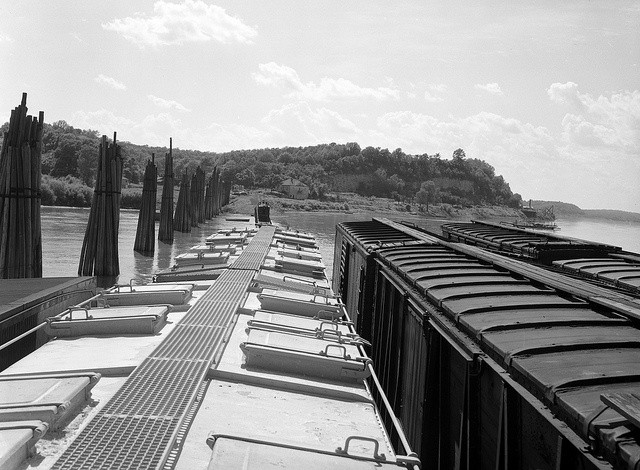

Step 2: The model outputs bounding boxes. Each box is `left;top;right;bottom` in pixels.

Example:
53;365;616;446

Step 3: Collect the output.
331;216;638;469
438;219;640;322
501;220;558;230
1;226;420;469
